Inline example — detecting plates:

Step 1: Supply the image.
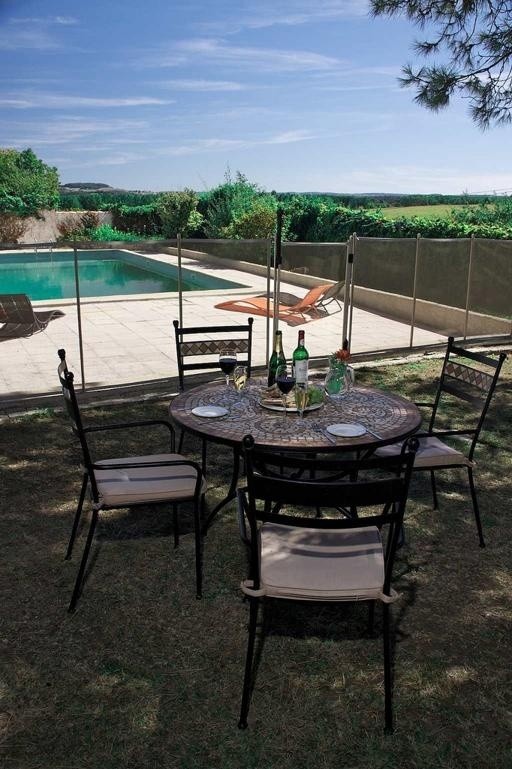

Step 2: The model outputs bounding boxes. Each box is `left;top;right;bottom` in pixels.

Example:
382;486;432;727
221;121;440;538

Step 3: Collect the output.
193;406;227;418
327;424;365;437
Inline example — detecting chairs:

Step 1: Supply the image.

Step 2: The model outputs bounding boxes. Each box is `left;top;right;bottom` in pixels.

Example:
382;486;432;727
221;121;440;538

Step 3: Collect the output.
214;280;348;327
1;293;64;338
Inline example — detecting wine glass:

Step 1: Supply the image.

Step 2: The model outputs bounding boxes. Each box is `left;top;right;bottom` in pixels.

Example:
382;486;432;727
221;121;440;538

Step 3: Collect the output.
219;349;237;389
275;364;294;418
294;382;306;423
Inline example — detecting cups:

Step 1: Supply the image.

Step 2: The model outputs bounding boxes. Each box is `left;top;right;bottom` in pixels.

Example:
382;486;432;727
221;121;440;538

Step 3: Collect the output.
232;366;248;389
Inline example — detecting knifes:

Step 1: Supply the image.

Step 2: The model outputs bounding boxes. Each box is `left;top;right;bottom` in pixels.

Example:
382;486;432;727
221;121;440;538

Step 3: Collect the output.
350;421;382;439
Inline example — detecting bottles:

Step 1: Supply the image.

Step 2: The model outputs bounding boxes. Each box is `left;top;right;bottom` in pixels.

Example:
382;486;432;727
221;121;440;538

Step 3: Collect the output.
268;330;287;388
292;330;309;388
